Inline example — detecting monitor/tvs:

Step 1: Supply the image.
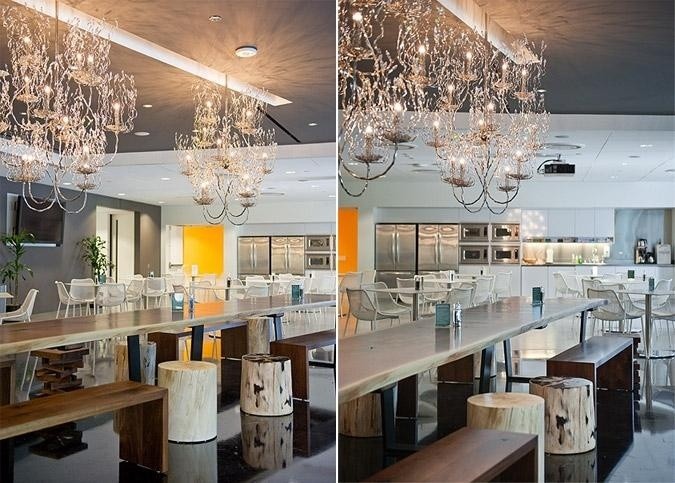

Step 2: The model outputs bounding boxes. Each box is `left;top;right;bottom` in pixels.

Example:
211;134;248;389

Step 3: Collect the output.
14;196;65;244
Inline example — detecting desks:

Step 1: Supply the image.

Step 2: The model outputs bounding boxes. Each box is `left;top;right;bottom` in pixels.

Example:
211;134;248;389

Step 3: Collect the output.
63;282;99;317
338;296;611;469
1;292;336;382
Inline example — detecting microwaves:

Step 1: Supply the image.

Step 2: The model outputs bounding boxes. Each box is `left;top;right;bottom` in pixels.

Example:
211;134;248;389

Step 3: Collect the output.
459;223;521;265
304;235;336;271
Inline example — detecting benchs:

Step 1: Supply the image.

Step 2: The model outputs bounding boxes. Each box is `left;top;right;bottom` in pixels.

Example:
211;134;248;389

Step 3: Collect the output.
546;336;635;406
1;381;170;482
269;330;336;457
147;319;249;400
594;390;635;483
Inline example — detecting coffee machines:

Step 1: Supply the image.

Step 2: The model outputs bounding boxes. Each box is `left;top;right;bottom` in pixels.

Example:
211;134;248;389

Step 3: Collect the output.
634;239;647;264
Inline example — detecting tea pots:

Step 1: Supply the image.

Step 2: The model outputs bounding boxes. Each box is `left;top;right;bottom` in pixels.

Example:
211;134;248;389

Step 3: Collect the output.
646;252;656;264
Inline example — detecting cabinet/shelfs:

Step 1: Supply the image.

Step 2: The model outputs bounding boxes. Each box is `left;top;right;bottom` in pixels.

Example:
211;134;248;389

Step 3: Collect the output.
459;207;521;224
521;209;576;239
575;207;614;239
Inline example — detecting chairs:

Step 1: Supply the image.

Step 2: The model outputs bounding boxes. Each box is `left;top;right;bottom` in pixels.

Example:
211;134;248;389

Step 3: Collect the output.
414;274;448;314
444;288;474;310
552;272;583;299
107;277;116;284
566;275;581;291
603;285;646;332
277;273;293;280
651;286;675;348
453;274;475;280
343;288;400;338
69;278;95;316
459;282;477;308
246;275;269;287
0;289;39;326
338;272;364;318
626;278;650;304
200;275;215;288
142;277;166;310
602;273;622;282
98;283;125;315
365;282;412;329
124;279;145;311
190;281;225;302
474;278;493;307
396;278;427;319
55;281;93;319
283;277;309;302
268;275;279;295
636;278;672;339
232;283;277;302
586;289;649;359
167;275;189;296
362;270;376;285
582;279;605;339
230;279;245;301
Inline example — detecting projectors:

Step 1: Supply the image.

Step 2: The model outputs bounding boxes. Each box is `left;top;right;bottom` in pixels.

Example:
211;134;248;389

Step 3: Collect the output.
543;164;576;176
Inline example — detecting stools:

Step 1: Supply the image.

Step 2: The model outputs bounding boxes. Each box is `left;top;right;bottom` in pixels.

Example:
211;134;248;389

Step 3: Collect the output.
166;438;220;483
156;361;218;444
544;449;597;482
114;341;157;387
465;392;547;483
241;411;294;471
529;376;597;455
111;411;122;435
338;392;383;438
240;353;295;417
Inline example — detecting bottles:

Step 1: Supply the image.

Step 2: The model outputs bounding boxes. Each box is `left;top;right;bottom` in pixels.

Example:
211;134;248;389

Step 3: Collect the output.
188;295;194;312
540;286;546;303
454;327;461;348
299;289;303;302
453;303;462;326
415;277;420;290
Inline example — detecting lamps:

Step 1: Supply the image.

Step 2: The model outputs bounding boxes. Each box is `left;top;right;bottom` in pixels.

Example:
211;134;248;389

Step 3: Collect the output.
338;0;426;197
1;2;138;212
426;1;547;213
172;75;277;226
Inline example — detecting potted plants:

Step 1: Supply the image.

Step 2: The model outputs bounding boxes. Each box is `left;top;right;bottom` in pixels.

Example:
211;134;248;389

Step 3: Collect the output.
1;227;35;323
74;235;116;309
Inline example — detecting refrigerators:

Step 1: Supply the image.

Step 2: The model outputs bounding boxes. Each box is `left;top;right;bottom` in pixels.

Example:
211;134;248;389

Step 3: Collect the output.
271;237;304;276
237;237;269;285
375;225;416;298
418;225;458;274
